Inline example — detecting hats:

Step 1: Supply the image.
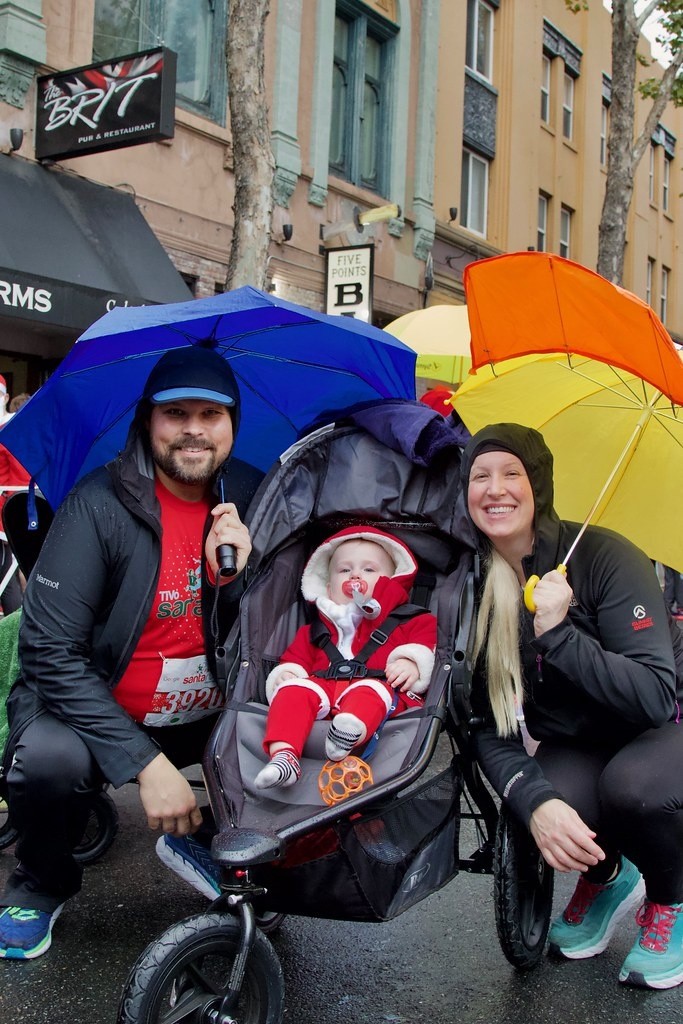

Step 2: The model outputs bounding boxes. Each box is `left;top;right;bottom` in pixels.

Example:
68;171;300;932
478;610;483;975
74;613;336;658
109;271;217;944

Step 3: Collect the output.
143;348;236;406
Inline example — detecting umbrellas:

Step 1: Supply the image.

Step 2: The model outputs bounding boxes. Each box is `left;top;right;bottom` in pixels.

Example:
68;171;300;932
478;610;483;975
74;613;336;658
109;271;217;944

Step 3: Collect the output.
0;285;419;575
382;304;472;384
443;251;683;613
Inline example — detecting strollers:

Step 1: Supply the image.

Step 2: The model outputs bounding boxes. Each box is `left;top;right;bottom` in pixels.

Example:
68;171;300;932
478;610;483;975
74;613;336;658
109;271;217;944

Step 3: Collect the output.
115;403;555;1024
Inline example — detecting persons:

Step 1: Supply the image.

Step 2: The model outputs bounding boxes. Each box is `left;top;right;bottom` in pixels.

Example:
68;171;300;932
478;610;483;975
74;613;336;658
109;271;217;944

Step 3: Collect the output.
0;345;277;959
460;423;683;989
662;564;683;619
0;375;39;615
253;524;437;789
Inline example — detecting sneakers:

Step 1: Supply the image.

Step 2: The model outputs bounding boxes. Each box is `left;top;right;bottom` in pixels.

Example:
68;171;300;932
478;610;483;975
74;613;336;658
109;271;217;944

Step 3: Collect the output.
0;904;64;959
618;898;683;989
155;831;224;902
547;857;646;959
